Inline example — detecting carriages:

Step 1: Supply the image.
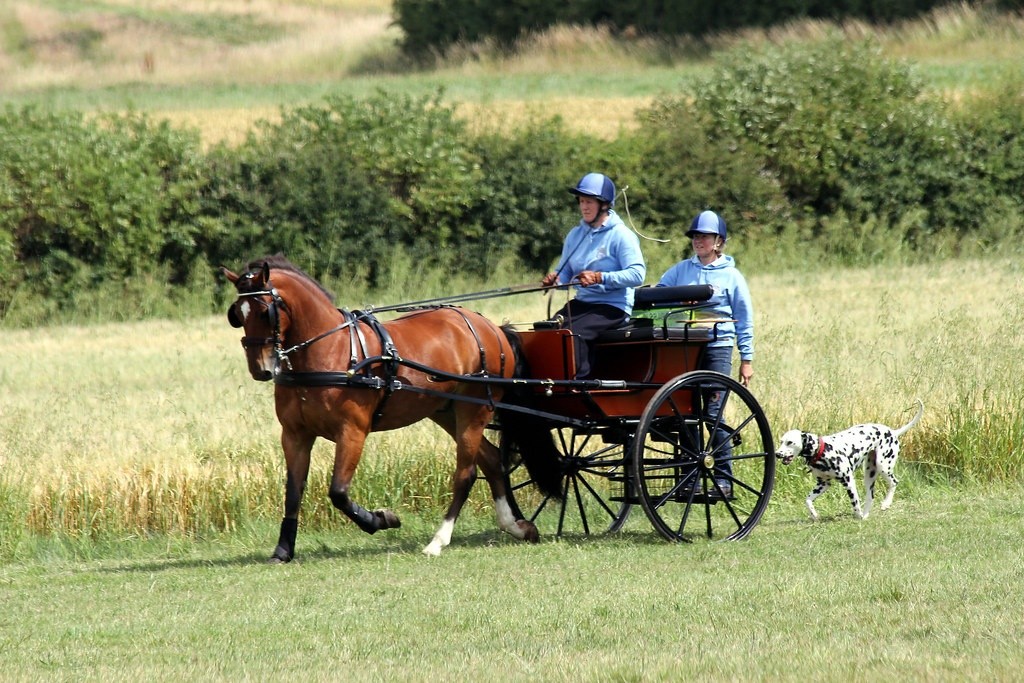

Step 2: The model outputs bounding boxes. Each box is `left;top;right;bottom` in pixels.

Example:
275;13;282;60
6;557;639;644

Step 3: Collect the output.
218;256;778;566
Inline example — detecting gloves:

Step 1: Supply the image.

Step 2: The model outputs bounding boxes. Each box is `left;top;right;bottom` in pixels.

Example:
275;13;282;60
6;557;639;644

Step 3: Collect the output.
574;270;602;288
540;274;556;288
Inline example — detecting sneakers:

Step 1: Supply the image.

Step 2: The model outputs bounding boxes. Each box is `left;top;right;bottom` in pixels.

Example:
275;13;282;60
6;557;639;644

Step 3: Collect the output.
708;479;733;497
665;474;701;497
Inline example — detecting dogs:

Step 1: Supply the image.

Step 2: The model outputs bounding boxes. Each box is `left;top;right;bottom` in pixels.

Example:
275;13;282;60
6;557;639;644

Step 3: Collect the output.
775;398;924;519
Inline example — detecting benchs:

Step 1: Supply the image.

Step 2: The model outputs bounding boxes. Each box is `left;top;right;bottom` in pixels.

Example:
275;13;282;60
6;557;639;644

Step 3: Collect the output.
588;285;725;392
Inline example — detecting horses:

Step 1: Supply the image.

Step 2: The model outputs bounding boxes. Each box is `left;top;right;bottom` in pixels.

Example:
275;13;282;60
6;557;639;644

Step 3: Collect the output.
213;256;568;563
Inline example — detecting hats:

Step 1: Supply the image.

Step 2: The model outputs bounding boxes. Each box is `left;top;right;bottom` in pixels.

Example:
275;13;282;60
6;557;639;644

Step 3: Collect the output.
564;172;616;204
685;210;727;240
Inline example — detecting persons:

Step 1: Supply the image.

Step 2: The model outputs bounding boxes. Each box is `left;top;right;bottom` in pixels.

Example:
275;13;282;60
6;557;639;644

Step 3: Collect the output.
655;213;753;498
543;173;647;388
